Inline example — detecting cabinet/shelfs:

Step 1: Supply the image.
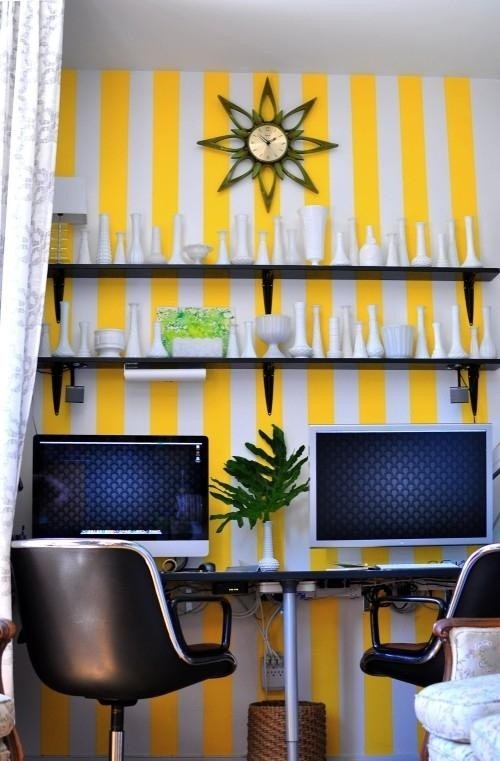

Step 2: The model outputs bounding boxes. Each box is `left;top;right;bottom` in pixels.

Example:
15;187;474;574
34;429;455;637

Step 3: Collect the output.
37;205;499;366
37;263;500;419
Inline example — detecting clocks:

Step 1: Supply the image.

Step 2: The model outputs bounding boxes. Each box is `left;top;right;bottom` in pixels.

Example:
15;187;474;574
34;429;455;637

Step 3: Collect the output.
247;122;291;166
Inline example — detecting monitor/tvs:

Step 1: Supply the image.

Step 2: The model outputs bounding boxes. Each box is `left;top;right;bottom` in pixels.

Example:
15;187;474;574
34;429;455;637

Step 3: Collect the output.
31;433;209;558
308;424;495;561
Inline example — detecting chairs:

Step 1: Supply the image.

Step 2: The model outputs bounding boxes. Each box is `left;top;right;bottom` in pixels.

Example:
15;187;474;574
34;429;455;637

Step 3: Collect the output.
415;615;499;760
10;537;236;761
0;618;25;761
358;543;500;687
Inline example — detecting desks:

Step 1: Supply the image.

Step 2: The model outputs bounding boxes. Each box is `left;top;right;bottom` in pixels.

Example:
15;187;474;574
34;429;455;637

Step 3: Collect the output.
155;563;463;761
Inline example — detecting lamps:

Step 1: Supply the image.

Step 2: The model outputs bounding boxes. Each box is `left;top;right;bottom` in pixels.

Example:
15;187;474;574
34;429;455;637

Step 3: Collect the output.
52;175;88;264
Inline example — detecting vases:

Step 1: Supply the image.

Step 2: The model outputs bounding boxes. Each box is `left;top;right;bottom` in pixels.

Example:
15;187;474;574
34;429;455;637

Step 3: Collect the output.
76;211;486;271
36;263;498;365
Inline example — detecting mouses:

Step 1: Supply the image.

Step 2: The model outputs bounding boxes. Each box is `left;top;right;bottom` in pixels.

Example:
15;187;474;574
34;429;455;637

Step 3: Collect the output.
200;561;215;573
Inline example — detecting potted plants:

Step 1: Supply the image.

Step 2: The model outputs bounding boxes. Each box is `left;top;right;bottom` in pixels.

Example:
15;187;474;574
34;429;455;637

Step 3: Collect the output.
205;421;312;572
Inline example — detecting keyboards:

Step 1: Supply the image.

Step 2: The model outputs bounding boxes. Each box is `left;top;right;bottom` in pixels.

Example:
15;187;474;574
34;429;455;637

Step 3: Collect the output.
376;562;458;571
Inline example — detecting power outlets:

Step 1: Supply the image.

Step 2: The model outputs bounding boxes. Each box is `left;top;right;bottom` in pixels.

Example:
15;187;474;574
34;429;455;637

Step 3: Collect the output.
261;655;285;691
